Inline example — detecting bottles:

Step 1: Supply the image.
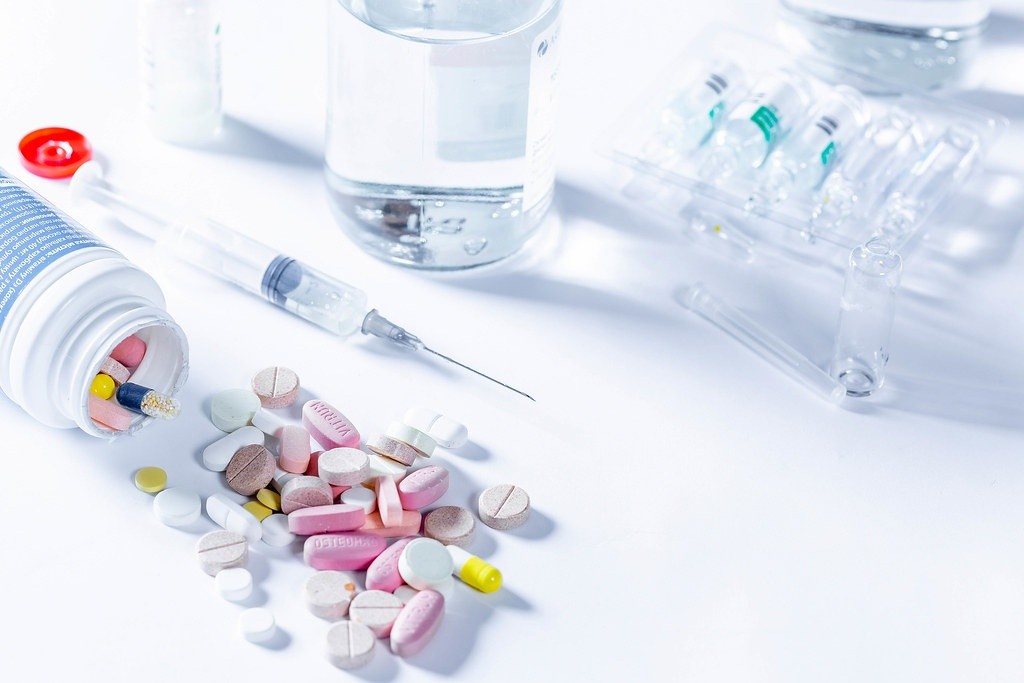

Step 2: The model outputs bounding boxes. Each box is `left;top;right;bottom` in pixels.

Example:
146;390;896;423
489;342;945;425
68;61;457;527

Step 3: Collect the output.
0;167;190;439
141;0;223;147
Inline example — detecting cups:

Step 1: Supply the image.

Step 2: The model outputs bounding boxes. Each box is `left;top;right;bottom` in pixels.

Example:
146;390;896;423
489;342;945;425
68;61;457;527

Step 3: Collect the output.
323;0;563;274
